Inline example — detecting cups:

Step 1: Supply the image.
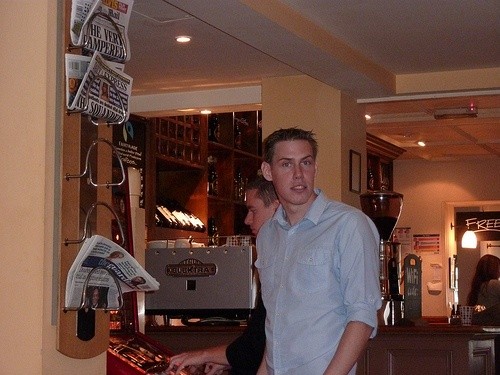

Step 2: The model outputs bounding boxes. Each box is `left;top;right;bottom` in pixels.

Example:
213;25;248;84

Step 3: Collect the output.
460;306;473;326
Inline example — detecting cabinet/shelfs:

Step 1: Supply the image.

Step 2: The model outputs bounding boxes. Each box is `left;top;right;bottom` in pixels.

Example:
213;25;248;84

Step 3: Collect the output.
146;325;500;374
147;110;404;304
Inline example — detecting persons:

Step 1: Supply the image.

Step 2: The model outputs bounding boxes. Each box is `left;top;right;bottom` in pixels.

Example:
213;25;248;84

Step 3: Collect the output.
468;254;500;314
86;288;104;308
166;179;280;375
108;250;124;261
254;128;382;375
130;276;146;287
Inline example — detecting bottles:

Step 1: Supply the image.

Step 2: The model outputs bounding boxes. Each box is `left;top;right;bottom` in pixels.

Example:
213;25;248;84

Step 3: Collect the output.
208;216;219;248
208;155;224;196
234;125;243;150
233;172;250;199
208;113;221;143
156;203;205;232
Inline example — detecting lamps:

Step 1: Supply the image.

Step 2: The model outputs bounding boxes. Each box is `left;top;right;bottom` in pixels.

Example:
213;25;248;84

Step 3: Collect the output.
451;222;480;249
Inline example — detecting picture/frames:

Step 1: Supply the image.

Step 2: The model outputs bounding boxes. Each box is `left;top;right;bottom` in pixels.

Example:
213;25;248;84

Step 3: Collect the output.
350;150;362;194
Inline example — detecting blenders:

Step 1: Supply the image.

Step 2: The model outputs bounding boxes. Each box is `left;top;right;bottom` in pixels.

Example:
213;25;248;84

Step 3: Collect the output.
361;192;405;299
